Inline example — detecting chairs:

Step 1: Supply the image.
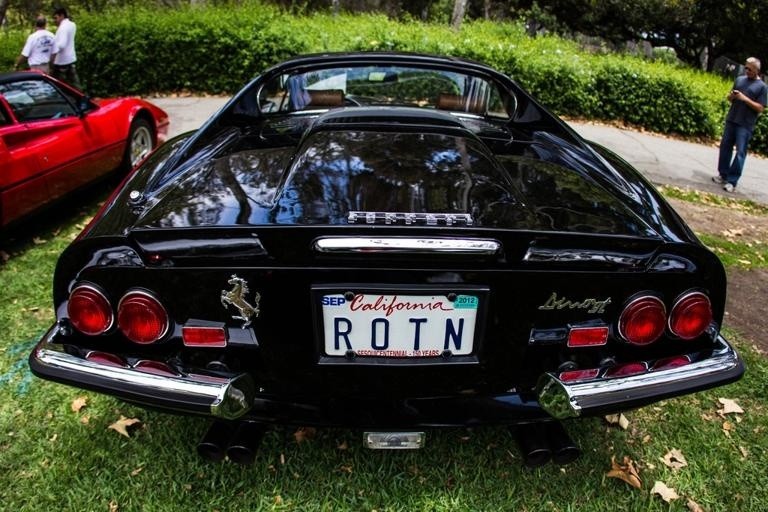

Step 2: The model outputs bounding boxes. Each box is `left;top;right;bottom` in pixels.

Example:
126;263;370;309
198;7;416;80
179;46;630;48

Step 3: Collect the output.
435;93;485;116
287;89;344;114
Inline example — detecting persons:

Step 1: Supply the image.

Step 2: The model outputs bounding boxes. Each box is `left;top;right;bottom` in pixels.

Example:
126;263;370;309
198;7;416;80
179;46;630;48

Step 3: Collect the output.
48;11;79;85
711;57;768;193
11;14;56;78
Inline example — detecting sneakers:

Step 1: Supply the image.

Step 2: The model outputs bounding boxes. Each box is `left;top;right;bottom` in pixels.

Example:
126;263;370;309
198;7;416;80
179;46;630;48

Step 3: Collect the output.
711;175;735;193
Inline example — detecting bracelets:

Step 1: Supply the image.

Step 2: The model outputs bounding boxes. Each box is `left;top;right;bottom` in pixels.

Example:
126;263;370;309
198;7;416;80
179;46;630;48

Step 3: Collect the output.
14;64;18;69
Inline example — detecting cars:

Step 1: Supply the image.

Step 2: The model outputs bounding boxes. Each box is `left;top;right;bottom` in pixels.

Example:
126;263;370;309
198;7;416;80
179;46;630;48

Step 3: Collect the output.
0;69;170;230
30;51;744;468
